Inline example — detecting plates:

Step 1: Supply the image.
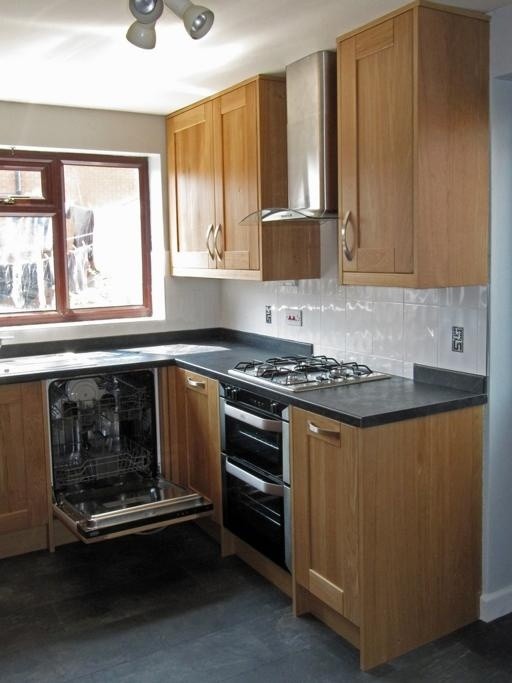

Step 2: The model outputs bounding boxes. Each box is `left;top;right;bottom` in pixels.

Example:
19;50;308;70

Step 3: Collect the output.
66;378;100;411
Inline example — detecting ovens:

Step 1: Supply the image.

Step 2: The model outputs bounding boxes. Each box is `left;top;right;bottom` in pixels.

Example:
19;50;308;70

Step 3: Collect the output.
221;396;292;575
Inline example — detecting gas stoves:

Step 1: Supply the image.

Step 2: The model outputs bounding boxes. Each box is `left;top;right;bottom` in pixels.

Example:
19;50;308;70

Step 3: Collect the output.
225;355;391;395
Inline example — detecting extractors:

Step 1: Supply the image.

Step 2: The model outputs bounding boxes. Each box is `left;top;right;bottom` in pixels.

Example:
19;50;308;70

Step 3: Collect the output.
237;50;338;227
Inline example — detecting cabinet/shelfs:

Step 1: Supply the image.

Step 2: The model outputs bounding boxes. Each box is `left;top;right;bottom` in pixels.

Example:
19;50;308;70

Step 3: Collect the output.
0;382;50;560
173;366;235;558
165;74;321;280
288;404;483;672
335;1;488;292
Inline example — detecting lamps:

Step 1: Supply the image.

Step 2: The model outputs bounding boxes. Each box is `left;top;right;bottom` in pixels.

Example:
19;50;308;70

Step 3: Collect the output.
125;0;215;50
